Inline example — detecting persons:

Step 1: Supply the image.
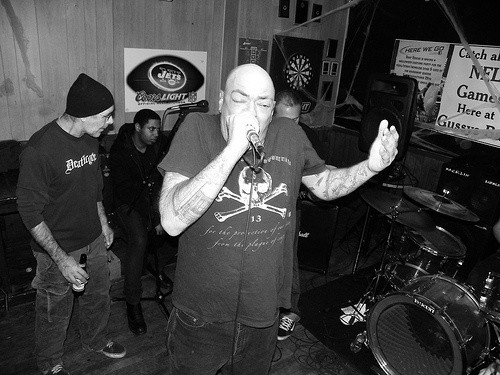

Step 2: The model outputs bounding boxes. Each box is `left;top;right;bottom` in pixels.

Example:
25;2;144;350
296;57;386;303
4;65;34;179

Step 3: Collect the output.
17;73;126;375
157;63;399;375
107;108;163;335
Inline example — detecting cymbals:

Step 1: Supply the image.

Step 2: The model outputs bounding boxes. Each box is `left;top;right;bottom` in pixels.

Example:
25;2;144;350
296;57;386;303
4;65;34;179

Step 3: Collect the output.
404;186;480;221
358;187;437;232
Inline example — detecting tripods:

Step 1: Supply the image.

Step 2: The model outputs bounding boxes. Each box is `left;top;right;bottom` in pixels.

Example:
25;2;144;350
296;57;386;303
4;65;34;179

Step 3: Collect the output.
111;108;190;322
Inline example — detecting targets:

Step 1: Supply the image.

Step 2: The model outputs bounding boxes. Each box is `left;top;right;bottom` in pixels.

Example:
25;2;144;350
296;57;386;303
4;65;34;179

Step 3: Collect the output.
283;52;313;91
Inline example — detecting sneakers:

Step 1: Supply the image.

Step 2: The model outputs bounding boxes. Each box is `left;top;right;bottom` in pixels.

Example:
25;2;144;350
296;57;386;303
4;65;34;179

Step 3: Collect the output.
43;364;69;375
277;316;296;340
98;340;126;359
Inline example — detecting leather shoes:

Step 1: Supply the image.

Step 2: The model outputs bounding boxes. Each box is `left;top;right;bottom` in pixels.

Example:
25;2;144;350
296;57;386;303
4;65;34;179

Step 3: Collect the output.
148;266;170;284
127;302;147;335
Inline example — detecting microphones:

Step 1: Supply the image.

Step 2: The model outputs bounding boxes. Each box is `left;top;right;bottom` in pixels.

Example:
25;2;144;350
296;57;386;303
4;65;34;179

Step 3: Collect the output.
167;100;208;110
246;126;266;159
404;167;419;187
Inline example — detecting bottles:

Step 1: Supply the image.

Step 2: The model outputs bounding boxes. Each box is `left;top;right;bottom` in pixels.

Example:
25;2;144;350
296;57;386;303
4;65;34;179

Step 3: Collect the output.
71;254;87;295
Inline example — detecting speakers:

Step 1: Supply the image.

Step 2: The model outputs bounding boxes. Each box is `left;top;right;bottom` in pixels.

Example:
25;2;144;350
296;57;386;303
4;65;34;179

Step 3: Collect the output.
297;196;344;276
354;74;460;198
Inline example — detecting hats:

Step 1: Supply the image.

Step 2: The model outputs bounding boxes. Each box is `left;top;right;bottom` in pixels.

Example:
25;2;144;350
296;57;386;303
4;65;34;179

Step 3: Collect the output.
66;72;114;118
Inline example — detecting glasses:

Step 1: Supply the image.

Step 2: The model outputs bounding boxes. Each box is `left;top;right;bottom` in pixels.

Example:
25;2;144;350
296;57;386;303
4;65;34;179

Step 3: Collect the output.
98;113;111;121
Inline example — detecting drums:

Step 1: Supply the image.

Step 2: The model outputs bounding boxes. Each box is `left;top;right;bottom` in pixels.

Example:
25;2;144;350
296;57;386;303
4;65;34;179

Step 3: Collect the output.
366;273;490;375
383;225;467;291
